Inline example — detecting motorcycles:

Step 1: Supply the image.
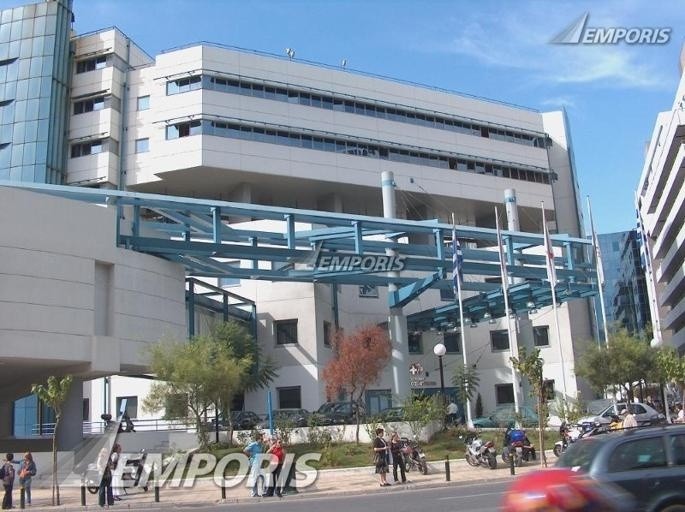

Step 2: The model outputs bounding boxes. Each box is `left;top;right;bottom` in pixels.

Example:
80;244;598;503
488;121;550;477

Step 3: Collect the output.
398;432;428;480
552;415;616;459
100;402;135;434
75;448;149;495
500;421;533;465
612;412;669;428
458;423;497;472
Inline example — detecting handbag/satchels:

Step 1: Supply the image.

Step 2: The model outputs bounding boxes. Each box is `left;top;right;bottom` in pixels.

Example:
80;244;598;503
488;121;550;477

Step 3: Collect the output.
19;469;23;480
0;465;3;479
371;453;386;466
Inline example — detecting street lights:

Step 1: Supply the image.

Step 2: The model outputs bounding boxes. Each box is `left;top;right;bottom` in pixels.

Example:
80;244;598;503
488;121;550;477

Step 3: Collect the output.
433;343;453;480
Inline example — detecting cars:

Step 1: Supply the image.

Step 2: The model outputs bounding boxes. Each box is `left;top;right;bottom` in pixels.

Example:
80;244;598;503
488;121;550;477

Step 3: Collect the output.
371;406;427;421
468;402;548;428
253;407;311;428
205;409;261;429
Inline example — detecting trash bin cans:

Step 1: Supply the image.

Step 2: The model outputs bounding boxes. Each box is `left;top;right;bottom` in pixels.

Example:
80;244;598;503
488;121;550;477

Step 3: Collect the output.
276;462;300;496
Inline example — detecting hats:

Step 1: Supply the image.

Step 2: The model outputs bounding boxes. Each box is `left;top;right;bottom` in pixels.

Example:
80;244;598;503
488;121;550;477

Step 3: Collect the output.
620;409;627;414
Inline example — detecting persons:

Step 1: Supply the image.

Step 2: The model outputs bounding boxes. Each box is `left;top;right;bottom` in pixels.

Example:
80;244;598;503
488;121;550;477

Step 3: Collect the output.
243;434;268;497
621;409;637;431
373;428;391;487
265;438;286;497
389;431;409;484
111;443;127;502
609;415;622;430
2;452;37;505
0;453;15;510
97;448;115;506
447;399;458;427
672;403;685;423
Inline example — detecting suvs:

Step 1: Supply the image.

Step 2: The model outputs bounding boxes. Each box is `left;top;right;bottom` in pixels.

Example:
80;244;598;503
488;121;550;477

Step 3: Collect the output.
546;424;684;511
576;402;668;431
312;399;366;424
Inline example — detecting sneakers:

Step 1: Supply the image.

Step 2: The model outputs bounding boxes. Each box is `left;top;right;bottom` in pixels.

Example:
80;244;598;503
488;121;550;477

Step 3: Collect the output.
402;480;411;484
252;494;260;497
393;481;400;485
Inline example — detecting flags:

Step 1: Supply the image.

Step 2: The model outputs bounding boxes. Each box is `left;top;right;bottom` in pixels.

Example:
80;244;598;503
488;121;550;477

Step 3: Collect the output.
452;230;464;295
499;227;510;291
592;212;604;285
545;219;558;288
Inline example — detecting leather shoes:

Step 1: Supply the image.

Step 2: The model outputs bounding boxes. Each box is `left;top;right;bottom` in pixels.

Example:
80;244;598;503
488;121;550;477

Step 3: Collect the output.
277;493;282;497
262;493;272;497
380;483;385;486
1;503;30;509
385;483;390;486
